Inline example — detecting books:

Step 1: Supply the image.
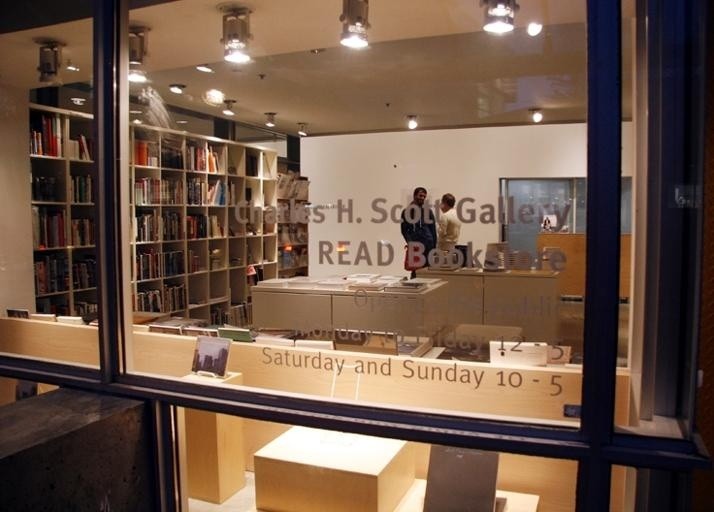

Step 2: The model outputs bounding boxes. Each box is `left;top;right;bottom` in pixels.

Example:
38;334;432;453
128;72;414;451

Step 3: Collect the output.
422;443;499;512
29;118;308;326
5;307;571;376
308;273;442;293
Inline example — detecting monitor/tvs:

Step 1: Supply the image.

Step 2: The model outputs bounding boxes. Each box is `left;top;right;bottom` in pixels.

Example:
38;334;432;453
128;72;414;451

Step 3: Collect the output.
453;243;470;268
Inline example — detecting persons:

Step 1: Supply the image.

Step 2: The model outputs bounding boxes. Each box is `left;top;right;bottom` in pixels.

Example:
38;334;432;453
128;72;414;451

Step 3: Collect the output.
401;187;437;278
438;193;462;251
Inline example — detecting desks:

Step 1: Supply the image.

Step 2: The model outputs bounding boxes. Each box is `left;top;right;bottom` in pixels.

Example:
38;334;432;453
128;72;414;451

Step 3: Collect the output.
132;311;170;324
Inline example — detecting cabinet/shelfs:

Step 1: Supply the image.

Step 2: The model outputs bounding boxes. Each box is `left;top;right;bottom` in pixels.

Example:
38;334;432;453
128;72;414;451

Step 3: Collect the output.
250;277;449;338
277;172;309;276
28;103;279;334
539;232;632;303
415;267;562;347
